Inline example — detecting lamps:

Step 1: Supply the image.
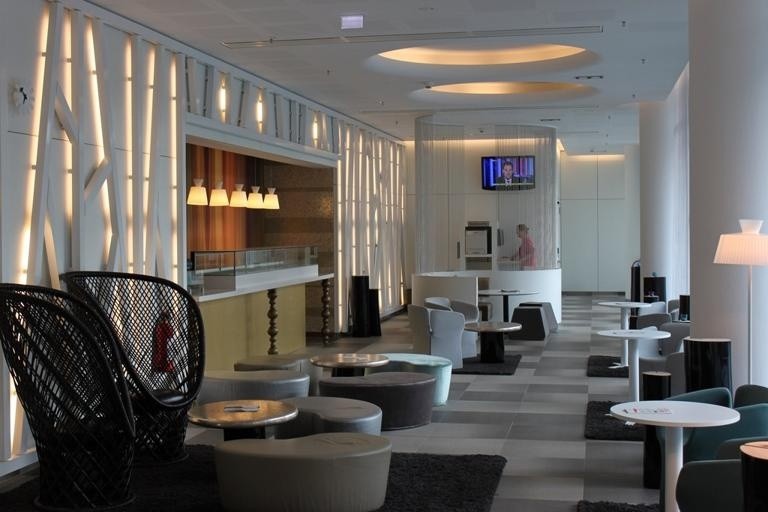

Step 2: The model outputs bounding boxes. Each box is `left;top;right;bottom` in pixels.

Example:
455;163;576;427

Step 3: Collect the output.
186;145;280;210
713;219;768;386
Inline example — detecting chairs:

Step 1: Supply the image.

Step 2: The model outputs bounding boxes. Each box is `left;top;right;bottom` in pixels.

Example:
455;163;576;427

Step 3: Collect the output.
58;270;205;467
424;297;478;358
637;298;691;395
0;282;137;512
405;304;465;370
659;384;768;508
676;436;768;512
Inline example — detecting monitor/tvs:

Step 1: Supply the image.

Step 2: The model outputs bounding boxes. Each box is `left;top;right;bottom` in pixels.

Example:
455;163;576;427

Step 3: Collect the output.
481;156;535;190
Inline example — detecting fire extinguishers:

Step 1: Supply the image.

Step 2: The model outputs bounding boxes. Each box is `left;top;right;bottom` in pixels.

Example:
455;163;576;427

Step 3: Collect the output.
151;311;176;372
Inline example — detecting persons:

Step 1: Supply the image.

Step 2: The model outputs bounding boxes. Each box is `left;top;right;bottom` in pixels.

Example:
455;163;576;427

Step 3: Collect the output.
503;223;535;270
495;161;519;190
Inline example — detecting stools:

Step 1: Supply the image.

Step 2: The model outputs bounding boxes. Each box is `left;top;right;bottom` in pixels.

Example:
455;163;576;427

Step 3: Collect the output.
508;306;550;341
519;302;558;335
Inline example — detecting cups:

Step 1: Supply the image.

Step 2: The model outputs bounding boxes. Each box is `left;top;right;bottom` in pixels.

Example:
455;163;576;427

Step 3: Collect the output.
649;291;655;297
680;314;688;322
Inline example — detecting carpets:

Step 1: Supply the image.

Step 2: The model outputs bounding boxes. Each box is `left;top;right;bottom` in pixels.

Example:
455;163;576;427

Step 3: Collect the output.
453;352;521;375
577;498;663;512
584;401;647;441
587;356;629;378
0;444;507;512
